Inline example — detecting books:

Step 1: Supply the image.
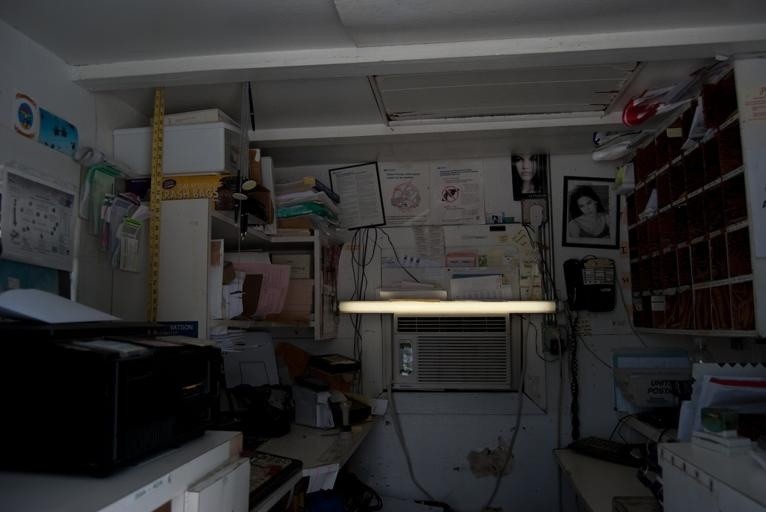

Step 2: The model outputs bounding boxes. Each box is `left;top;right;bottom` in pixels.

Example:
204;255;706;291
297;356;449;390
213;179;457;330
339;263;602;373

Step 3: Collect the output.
247;146;344;236
375;280;453;299
691;422;759;455
221;264;314;315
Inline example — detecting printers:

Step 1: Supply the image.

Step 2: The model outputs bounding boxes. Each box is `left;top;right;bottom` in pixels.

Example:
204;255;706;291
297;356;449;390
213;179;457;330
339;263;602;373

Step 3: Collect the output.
0;287;209;480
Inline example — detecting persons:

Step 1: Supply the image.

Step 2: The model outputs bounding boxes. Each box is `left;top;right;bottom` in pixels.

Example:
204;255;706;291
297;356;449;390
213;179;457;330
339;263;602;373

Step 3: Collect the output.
511;153;548;199
568;184;612;240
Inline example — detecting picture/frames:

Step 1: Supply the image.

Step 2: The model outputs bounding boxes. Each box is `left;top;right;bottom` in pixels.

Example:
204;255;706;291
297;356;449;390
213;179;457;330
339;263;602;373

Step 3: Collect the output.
561;174;621;250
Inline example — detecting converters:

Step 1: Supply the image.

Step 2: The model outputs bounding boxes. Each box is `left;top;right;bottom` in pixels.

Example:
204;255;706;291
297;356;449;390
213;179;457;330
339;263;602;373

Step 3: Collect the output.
549;338;565;354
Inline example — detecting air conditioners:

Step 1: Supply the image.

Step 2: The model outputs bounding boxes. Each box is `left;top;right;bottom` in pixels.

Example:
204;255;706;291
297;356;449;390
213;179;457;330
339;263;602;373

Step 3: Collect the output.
390;313;512;390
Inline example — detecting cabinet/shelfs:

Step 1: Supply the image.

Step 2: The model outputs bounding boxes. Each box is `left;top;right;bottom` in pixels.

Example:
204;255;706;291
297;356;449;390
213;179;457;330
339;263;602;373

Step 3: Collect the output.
624;59;765;339
4;429;250;512
156;196;338;343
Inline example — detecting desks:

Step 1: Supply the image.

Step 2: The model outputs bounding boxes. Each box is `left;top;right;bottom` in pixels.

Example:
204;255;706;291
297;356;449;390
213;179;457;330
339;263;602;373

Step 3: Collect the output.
234;416;373;511
553;447;665;511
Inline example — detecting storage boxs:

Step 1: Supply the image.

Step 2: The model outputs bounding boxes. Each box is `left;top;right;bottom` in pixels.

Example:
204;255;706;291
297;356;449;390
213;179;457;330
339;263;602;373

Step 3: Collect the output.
113;122;243;177
